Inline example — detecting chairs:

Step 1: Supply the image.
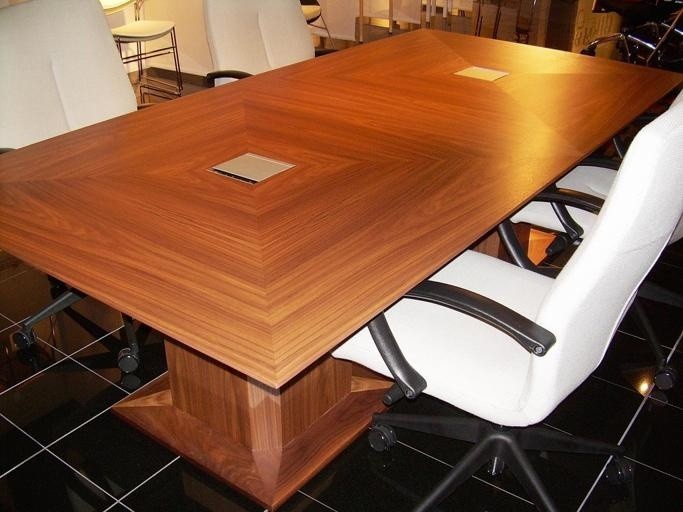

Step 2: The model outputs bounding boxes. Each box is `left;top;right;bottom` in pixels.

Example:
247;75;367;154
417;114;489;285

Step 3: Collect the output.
203;1;317;88
1;1;144;375
101;0;185;109
331;82;683;511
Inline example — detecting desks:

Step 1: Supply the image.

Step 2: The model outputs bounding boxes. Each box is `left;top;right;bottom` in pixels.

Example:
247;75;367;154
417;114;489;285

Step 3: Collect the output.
0;28;683;512
